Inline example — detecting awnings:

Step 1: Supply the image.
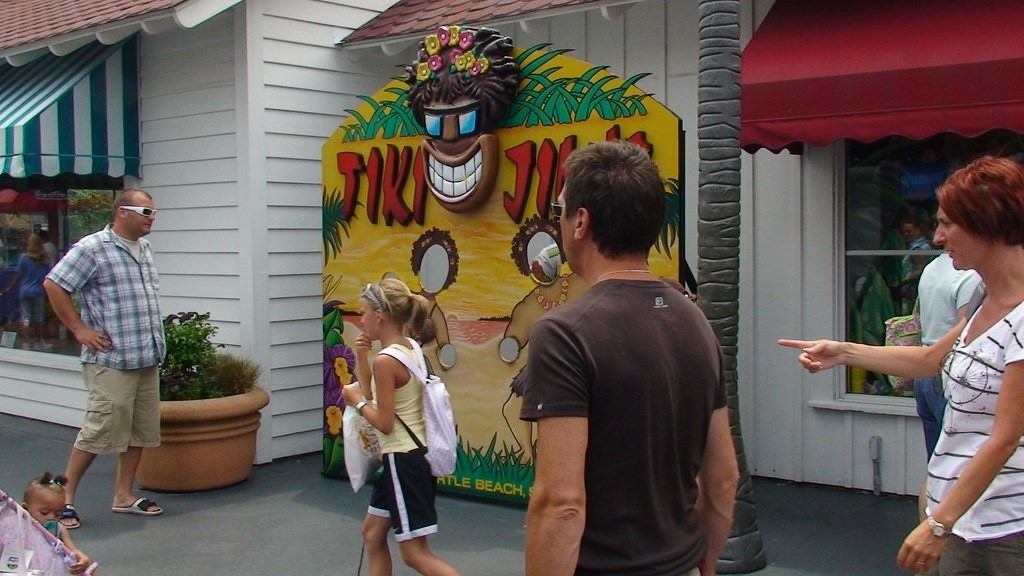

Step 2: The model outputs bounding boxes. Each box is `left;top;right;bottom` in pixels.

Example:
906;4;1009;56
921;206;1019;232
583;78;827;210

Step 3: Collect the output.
0;32;139;178
741;0;1024;155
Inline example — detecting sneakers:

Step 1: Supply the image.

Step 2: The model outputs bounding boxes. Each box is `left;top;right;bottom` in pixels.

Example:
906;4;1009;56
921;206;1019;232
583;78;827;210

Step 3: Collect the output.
22;337;54;350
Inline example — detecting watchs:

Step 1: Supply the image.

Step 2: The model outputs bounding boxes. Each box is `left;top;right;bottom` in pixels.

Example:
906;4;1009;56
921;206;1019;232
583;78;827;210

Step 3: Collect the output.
927;516;950;538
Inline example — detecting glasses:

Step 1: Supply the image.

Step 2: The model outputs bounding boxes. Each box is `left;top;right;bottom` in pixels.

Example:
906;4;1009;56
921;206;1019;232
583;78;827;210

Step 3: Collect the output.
550;202;565;217
362;282;381;308
118;206;157;220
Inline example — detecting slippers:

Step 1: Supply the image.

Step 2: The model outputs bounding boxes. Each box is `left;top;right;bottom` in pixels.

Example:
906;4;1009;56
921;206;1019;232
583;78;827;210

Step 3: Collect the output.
60;503;80;530
112;496;163;516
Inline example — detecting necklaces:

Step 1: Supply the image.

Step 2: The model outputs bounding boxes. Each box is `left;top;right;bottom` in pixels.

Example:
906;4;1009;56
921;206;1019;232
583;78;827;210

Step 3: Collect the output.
596;269;651;280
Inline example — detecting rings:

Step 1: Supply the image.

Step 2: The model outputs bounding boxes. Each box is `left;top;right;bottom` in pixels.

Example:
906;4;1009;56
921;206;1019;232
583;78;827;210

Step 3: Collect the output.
915;561;925;567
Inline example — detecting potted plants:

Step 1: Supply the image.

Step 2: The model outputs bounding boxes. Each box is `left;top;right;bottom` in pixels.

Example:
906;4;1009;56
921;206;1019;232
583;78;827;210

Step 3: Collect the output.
135;312;270;492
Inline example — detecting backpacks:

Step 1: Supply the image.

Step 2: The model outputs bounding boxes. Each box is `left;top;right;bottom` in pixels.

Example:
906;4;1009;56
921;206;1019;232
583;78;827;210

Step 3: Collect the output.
377;337;457;478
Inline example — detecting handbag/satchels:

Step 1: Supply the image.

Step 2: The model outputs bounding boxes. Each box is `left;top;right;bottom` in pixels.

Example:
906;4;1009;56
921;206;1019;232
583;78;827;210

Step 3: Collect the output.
342;382;382;493
884;295;920;392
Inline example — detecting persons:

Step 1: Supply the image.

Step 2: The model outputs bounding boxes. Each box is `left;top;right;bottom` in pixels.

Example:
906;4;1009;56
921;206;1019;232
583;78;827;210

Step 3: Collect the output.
518;143;738;575
43;188;167;528
21;472;98;576
342;278;462;576
778;157;1024;576
0;234;59;343
